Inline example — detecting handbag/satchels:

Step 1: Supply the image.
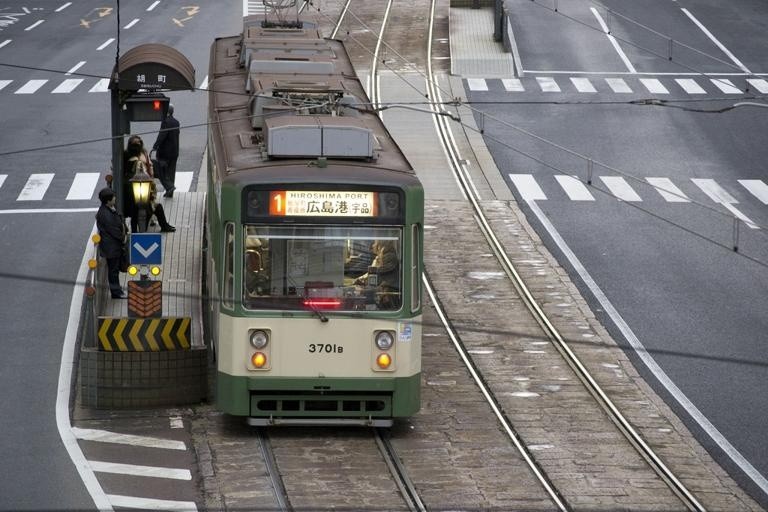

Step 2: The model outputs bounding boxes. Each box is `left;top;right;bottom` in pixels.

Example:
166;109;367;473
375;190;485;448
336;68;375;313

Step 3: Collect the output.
149;149;168;178
142;149;152;177
119;246;129;273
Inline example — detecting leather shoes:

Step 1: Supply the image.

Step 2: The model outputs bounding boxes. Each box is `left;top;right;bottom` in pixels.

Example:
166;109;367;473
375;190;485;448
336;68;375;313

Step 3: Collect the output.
163;187;176;197
119;293;127;298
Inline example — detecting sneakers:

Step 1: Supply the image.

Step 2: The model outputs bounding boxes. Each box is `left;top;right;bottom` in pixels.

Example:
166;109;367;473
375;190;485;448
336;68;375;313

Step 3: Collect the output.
160;223;176;232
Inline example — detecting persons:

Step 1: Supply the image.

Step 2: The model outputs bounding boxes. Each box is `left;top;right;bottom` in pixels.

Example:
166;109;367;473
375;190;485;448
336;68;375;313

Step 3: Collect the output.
94;186;130;300
227;223;270;296
120;135;155;198
121;158;177;233
151;103;180;198
343;239;402;310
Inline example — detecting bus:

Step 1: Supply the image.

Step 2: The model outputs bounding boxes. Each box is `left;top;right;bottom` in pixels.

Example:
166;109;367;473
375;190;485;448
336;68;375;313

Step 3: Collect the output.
201;12;425;444
